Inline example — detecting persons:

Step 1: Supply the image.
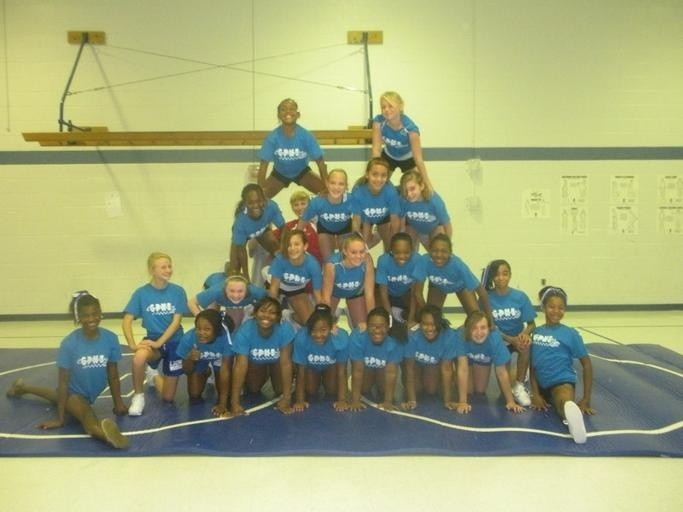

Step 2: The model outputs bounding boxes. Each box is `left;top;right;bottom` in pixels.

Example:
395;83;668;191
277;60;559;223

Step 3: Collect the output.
371;90;434;195
123;251;188;416
177;154;538;417
530;286;595;445
256;97;329;199
7;288;129;449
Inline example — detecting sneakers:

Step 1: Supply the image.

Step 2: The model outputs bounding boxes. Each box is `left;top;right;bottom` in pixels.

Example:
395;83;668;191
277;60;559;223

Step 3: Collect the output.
145;365;158;388
4;377;24;399
562;400;588;445
101;416;129;450
128;391;146;417
513;381;531;407
